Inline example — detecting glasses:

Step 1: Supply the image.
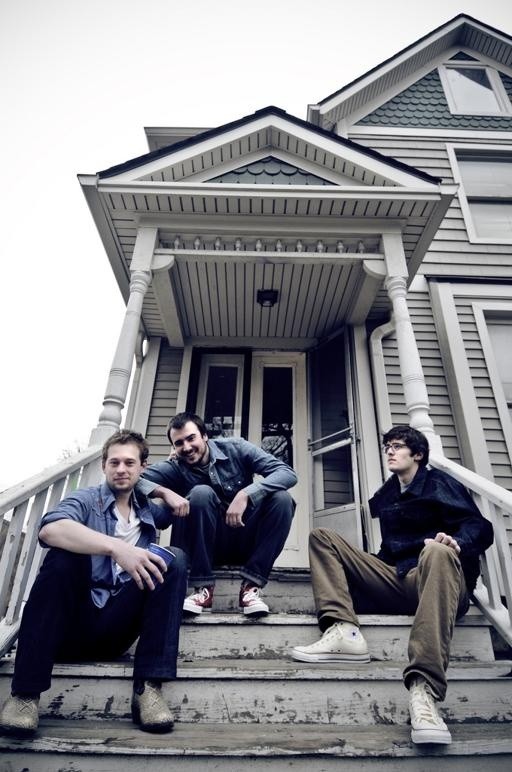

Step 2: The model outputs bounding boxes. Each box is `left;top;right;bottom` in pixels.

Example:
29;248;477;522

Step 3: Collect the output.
383;444;406;452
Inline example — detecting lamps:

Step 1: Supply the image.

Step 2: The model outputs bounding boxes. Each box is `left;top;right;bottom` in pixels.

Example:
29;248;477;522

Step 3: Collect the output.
256;286;281;309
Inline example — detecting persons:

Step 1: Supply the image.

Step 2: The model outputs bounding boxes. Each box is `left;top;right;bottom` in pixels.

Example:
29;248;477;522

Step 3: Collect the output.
134;412;298;616
0;430;189;734
291;425;494;745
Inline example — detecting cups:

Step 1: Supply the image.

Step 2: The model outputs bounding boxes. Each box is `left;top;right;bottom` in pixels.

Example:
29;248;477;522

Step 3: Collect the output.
136;542;177;590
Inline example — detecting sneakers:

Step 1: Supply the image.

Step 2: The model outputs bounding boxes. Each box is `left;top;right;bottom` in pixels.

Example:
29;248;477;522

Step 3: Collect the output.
131;681;173;732
1;693;39;734
291;621;371;664
181;586;215;613
407;676;453;745
239;578;269;616
